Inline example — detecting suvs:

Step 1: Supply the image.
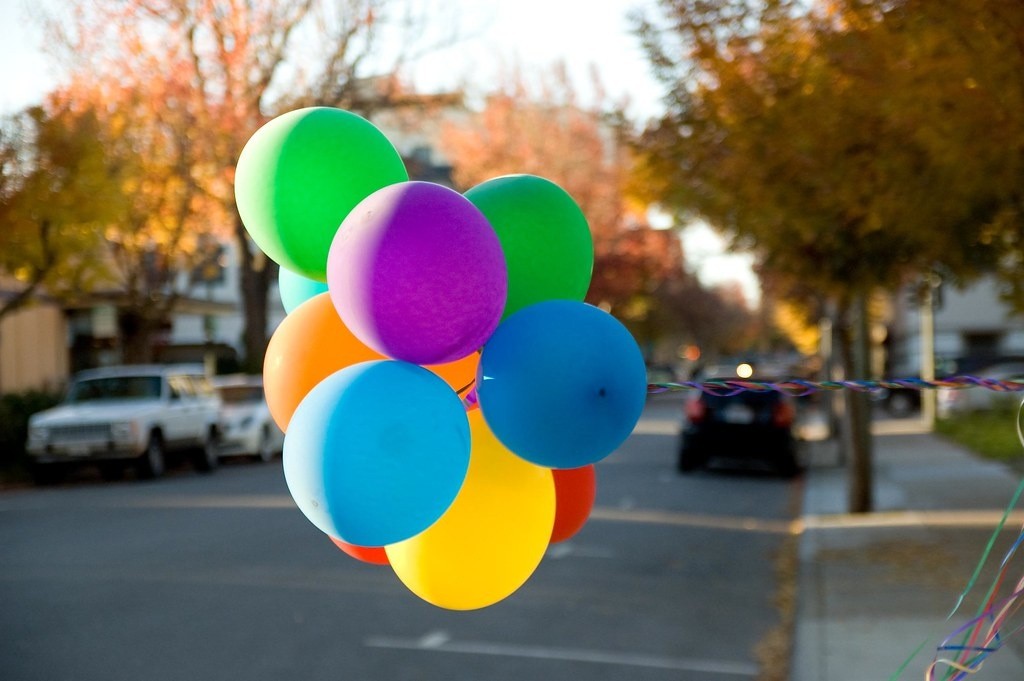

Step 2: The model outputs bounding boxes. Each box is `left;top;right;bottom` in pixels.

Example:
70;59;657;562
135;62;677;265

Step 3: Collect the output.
27;363;227;487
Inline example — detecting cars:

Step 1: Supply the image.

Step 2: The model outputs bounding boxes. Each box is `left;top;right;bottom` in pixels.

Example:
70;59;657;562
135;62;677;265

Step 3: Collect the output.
873;354;1024;422
678;371;798;480
211;372;287;463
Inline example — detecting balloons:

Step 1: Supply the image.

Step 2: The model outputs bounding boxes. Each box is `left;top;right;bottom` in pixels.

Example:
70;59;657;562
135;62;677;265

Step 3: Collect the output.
235;107;647;611
